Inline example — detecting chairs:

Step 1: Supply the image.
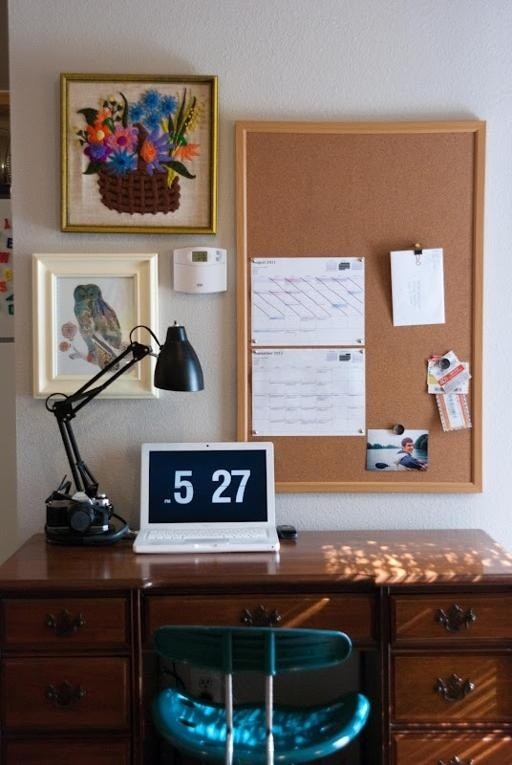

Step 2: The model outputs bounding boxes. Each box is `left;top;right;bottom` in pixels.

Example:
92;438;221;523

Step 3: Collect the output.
149;622;372;763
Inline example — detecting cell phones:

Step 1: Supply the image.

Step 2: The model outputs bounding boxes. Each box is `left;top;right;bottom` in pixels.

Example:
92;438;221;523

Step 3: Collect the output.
277;524;298;539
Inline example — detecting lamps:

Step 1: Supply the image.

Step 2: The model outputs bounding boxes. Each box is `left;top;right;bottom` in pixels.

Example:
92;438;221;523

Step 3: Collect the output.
36;326;204;548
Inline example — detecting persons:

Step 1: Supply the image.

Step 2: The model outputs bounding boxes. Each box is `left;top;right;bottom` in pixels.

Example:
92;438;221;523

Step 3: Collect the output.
392;437;427;471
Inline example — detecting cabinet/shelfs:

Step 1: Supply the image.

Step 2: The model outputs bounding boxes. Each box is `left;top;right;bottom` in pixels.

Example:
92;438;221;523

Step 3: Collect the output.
0;530;511;764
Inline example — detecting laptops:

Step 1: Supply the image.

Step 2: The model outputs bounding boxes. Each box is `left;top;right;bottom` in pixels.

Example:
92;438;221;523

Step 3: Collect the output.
130;441;282;557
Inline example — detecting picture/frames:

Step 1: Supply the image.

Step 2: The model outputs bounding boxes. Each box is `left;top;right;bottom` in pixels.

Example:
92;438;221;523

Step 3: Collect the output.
233;122;485;496
30;251;158;403
60;72;219;234
